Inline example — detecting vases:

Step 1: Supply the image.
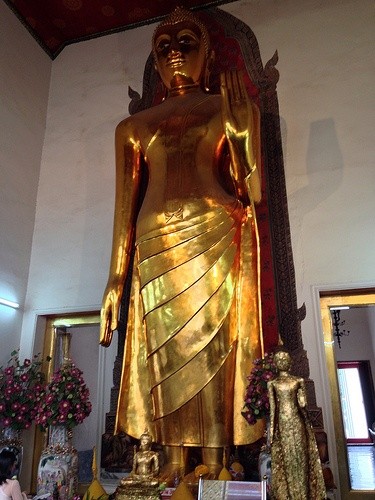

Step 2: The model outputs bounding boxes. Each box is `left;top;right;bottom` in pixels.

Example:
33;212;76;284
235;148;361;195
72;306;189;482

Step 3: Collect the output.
260;426;278;495
36;425;81;500
0;426;27;483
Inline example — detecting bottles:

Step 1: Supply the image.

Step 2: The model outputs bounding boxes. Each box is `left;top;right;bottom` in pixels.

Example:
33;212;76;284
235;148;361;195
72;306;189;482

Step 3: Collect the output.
173;471;180;487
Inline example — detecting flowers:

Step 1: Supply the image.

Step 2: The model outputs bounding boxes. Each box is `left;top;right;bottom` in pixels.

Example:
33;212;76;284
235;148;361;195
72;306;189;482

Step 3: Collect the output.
0;350;44;433
33;360;94;428
242;356;282;425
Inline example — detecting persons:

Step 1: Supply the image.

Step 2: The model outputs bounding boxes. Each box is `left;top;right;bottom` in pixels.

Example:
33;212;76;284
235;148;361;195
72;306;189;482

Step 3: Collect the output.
265;350;329;500
119;433;160;487
97;2;271;493
0;448;28;500
51;479;69;500
